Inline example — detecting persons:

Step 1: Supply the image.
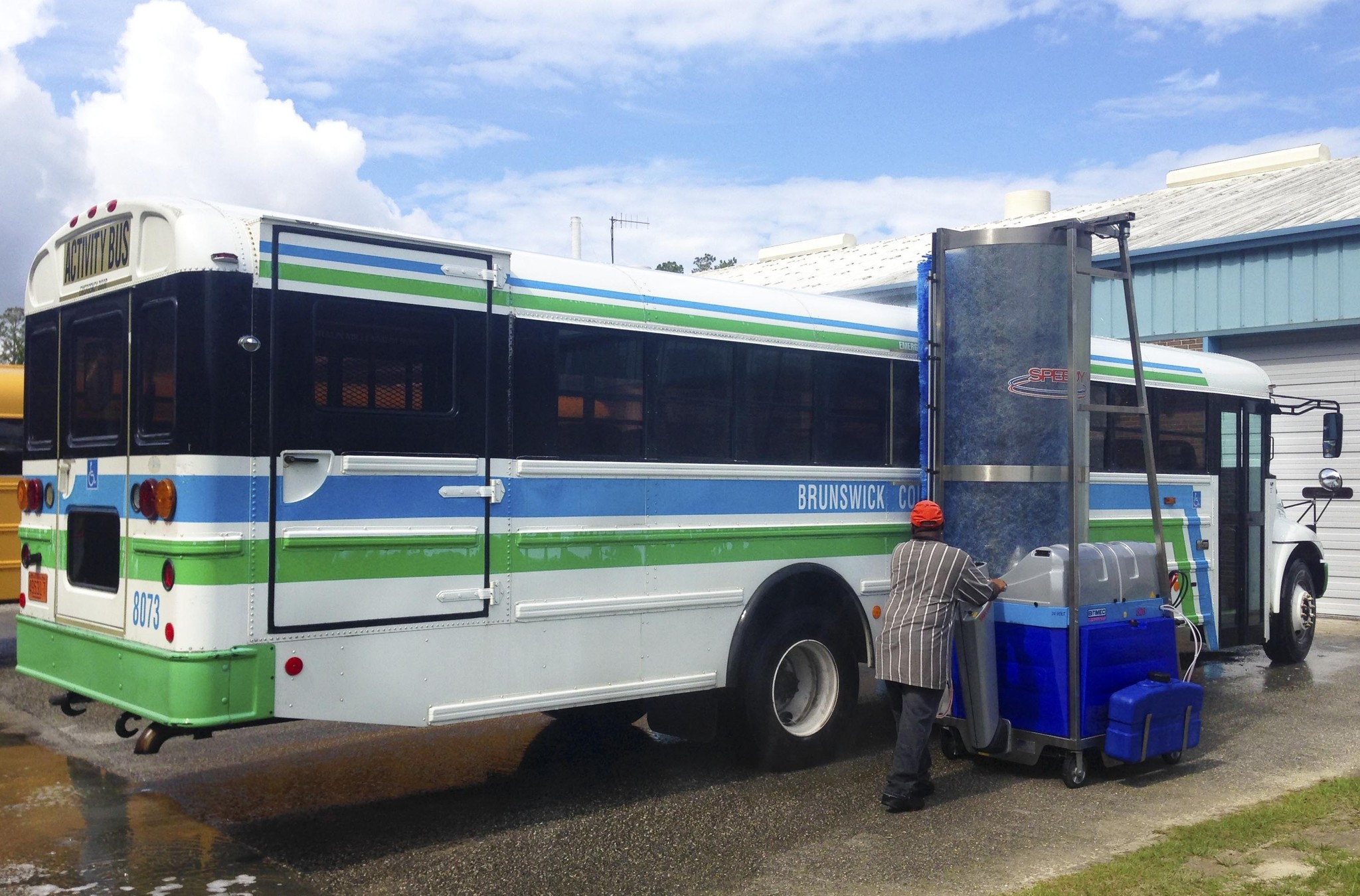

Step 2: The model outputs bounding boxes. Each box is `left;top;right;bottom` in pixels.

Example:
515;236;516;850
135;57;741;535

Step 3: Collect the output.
875;500;1008;812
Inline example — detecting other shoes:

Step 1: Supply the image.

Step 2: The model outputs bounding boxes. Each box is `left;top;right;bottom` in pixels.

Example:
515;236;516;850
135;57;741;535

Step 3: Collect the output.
881;793;925;811
917;782;932;791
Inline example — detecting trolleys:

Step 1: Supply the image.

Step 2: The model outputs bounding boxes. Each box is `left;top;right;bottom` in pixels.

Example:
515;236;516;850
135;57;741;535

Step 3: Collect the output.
917;212;1205;789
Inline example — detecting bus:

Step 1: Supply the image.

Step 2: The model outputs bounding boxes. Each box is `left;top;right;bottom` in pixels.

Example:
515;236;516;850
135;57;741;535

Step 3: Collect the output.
0;198;1354;774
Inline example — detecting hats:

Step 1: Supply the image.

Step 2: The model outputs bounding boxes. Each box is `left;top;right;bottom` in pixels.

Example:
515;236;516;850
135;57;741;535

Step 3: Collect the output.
910;500;944;528
